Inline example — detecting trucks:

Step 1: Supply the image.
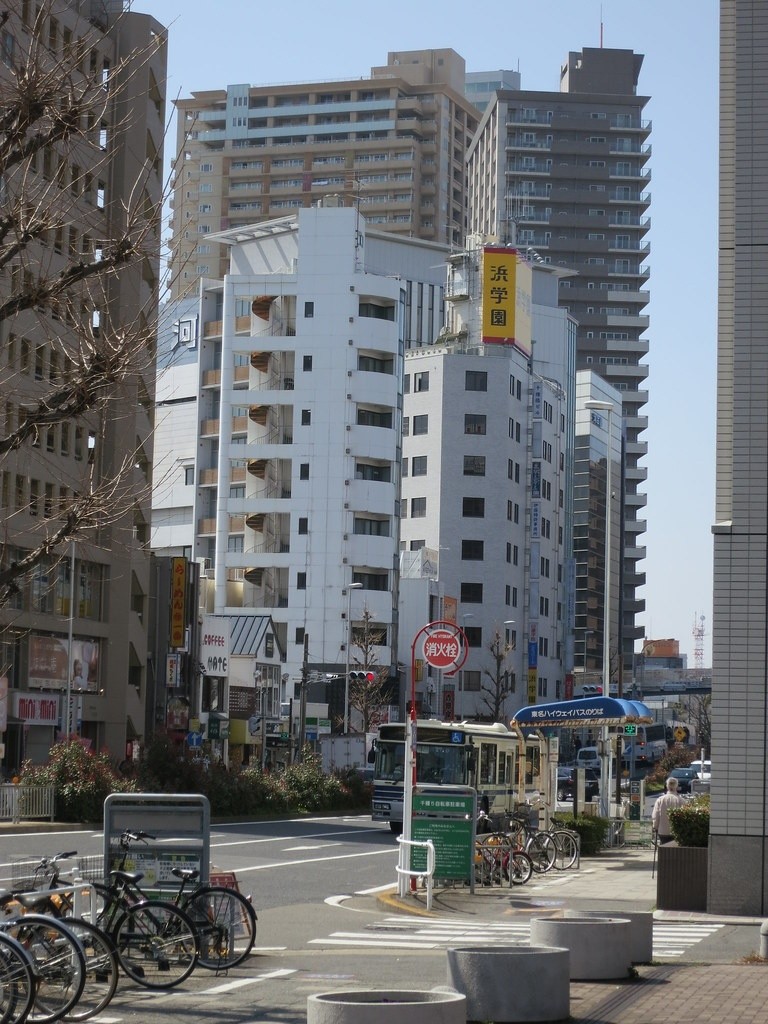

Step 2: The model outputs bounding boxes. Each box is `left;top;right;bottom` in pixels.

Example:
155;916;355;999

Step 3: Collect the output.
621;720;698;766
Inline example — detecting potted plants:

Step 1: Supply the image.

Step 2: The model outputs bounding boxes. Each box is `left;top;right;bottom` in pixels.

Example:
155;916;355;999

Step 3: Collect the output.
657;806;709;912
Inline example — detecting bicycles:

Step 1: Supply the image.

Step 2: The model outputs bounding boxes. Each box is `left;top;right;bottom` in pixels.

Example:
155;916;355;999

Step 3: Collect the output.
0;831;260;1024
475;791;579;884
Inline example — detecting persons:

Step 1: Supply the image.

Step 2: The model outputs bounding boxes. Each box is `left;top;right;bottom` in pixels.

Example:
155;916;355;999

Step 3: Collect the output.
652;776;687;844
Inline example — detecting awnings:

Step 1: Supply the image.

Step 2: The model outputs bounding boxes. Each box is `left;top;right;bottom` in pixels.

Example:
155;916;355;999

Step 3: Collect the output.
509;697;652;725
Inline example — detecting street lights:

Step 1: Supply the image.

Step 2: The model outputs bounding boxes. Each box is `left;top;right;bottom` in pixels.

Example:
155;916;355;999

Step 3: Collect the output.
580;630;595;701
460;611;474;723
631;638;675;701
500;619;516;724
343;582;363;735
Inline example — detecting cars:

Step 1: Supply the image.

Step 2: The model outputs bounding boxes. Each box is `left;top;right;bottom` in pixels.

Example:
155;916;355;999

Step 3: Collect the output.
575;745;603;772
665;769;700;794
689;760;711;785
557;766;598;803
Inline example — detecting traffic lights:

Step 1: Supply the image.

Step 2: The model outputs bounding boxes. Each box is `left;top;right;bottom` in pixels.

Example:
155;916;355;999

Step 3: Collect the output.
347;671;378;685
582;685;603;694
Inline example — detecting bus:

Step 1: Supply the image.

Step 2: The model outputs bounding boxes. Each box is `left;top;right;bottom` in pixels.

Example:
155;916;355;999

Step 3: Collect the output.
367;718;544;834
